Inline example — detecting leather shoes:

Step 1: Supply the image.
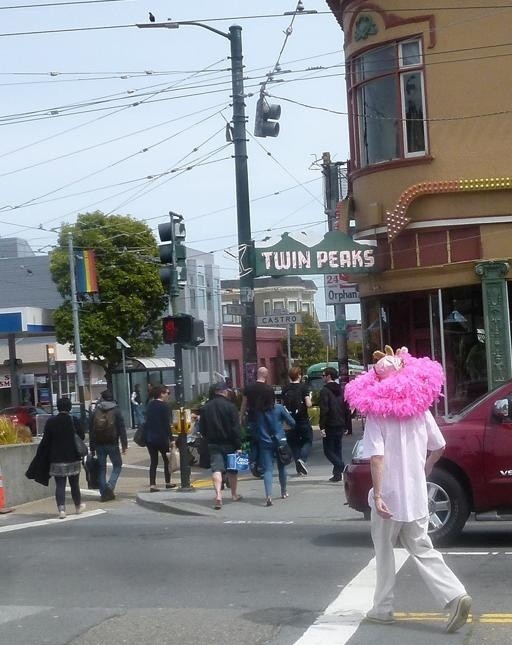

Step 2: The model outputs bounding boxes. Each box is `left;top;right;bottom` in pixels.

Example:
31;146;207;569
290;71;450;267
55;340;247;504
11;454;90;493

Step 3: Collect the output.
150;487;160;492
166;484;176;488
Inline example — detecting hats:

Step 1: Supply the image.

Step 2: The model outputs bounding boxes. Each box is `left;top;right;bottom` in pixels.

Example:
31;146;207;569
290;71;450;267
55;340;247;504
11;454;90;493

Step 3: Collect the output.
214;382;232;392
101;390;113;401
372;345;406;379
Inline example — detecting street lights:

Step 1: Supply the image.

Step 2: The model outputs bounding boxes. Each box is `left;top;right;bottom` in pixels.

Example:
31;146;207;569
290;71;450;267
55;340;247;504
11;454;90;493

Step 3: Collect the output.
136;16;258;393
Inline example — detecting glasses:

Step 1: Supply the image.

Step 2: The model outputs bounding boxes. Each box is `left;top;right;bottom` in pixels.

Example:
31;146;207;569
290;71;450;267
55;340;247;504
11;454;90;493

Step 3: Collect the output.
165;391;171;396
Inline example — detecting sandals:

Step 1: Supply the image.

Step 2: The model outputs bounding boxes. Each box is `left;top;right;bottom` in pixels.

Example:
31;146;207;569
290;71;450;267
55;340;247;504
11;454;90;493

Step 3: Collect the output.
265;497;273;507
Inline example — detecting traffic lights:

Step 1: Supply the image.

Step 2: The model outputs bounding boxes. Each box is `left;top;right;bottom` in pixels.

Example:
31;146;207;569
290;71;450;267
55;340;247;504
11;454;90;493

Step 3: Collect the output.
162;316;193;345
253;93;282;139
158;223;175;287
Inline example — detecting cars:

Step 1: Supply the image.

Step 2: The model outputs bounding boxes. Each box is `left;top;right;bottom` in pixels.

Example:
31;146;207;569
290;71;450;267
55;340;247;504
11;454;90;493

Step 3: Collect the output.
1;406;49;437
53;408;90;427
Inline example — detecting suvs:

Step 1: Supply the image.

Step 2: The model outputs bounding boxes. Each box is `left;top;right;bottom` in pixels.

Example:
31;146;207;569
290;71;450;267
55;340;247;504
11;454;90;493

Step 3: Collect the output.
341;379;512;548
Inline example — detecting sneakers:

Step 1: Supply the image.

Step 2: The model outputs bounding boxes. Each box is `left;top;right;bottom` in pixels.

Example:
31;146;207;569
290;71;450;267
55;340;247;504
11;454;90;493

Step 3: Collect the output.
444;594;472;635
296;459;309;477
328;473;342;482
75;503;86;514
367;607;396;624
99;485;115;501
51;504;68;519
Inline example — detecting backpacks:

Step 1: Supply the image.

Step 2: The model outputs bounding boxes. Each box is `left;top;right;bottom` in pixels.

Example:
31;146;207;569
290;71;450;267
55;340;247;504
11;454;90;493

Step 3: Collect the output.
283;387;305;421
91;408;118;445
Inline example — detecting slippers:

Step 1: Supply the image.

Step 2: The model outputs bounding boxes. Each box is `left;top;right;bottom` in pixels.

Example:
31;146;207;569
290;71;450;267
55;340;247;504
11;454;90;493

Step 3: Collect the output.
215;496;223;510
281;492;289;499
231;495;242;502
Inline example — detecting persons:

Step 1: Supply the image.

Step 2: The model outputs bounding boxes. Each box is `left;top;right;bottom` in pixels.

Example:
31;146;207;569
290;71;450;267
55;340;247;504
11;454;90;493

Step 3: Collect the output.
42;398;88;520
131;384;143;429
145;383;155;404
318;368;351;482
88;389;128;501
184;360;313;510
144;385;176;492
342;346;474;634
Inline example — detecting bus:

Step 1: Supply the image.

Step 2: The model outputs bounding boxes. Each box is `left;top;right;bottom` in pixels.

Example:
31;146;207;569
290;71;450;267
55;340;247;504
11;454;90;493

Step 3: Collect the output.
307;360;363;393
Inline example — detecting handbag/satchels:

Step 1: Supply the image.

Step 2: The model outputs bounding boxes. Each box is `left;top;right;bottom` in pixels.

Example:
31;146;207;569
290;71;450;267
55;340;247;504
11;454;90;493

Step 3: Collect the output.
74;433;89;455
83;454;102;490
275;442;294;465
